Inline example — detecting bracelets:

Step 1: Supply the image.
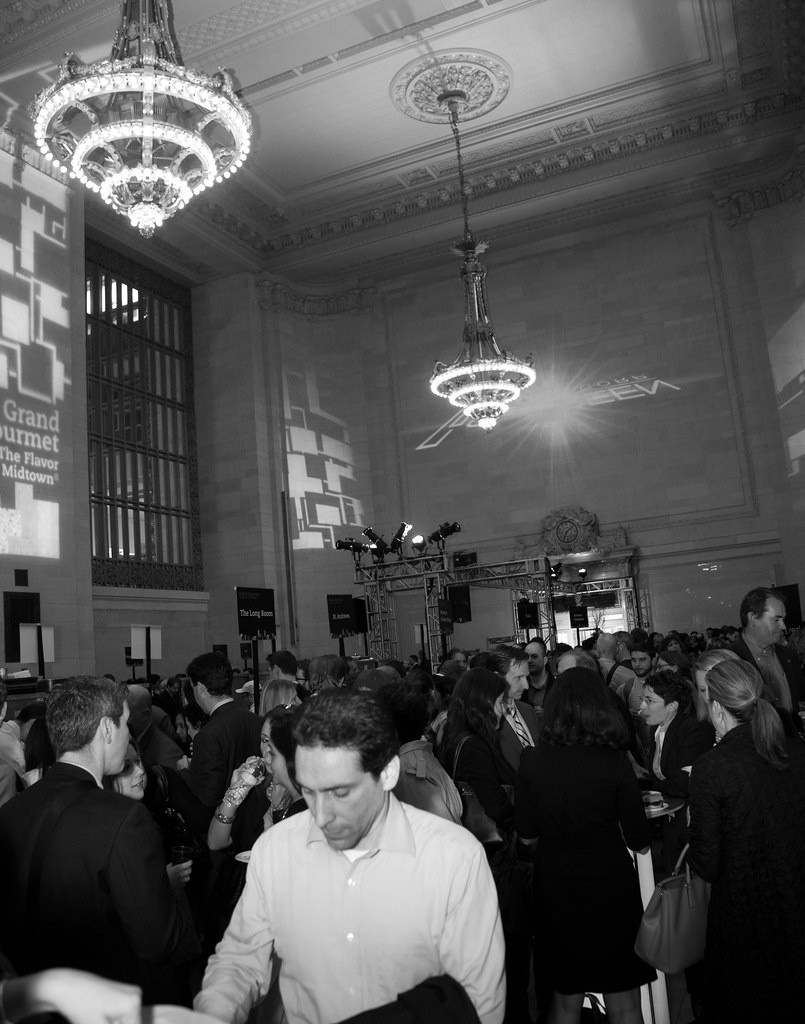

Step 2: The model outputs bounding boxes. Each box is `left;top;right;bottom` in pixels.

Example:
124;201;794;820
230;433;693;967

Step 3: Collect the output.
214;806;235;824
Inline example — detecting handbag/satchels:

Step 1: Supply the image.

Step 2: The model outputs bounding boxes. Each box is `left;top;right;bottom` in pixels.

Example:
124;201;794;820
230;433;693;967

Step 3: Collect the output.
633;842;712;974
488;829;535;940
153;764;189;842
446;735;515;845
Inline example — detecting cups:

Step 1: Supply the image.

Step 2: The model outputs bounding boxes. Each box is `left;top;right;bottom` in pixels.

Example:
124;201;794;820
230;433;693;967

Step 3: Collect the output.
172;846;193;866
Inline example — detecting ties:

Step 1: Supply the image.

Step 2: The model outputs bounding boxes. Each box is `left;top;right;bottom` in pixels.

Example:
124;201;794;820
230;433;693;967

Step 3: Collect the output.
505;704;531;748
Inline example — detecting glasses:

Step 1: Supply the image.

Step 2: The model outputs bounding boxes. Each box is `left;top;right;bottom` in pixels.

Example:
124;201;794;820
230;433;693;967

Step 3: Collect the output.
654;664;672;669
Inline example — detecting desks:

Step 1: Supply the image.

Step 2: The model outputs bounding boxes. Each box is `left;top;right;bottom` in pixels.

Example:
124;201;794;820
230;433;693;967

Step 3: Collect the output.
2;692;52;722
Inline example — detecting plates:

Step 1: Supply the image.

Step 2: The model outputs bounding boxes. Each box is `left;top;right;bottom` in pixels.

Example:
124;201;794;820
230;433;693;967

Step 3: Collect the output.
235;851;251;863
641;793;687;818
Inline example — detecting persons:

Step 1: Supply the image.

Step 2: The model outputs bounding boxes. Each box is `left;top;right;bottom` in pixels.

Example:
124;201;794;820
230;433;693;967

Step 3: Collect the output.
0;587;805;1024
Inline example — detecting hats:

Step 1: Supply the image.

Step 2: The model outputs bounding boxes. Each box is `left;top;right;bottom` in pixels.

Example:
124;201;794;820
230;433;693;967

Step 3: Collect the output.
235;680;262;695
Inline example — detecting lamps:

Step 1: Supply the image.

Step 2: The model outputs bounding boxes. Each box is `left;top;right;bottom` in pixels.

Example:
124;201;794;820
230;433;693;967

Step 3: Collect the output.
24;0;252;240
550;562;588;582
334;521;461;562
427;90;535;433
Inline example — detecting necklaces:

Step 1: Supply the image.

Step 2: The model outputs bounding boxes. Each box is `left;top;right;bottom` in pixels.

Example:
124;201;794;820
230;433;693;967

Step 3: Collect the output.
265;780;287;814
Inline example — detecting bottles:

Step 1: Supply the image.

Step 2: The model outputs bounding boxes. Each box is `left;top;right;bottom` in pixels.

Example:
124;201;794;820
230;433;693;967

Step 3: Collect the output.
223;758;265;809
